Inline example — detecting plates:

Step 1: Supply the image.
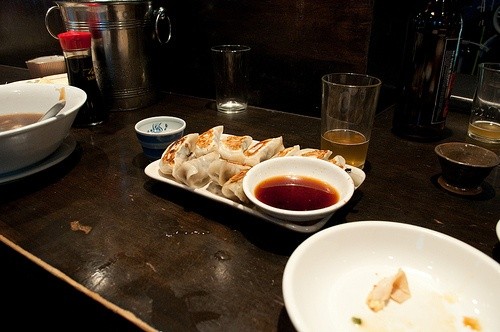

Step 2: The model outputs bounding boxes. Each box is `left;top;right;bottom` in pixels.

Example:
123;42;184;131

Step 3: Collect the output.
11;73;68;83
143;134;366;233
282;220;500;332
0;133;79;183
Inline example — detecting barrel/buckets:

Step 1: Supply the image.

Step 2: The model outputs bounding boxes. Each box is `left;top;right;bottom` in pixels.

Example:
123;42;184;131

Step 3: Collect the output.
45;0;172;113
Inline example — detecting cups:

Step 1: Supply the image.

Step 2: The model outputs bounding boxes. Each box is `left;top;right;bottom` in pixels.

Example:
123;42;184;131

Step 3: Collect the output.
467;62;500;144
434;143;500;195
211;44;251;114
320;73;381;169
25;54;67;78
135;116;186;162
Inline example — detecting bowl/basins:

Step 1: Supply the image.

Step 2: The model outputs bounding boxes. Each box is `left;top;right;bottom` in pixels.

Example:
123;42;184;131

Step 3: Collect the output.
244;155;355;221
0;83;87;174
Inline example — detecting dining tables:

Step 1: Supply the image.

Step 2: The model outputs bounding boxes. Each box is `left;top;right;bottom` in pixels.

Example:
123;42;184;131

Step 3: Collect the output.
0;62;500;332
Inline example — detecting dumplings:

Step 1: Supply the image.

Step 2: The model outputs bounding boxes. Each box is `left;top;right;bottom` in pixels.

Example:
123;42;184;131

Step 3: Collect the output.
158;125;348;204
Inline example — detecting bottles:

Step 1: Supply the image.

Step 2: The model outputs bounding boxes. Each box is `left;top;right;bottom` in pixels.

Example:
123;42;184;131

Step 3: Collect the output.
392;0;463;143
56;31;109;125
